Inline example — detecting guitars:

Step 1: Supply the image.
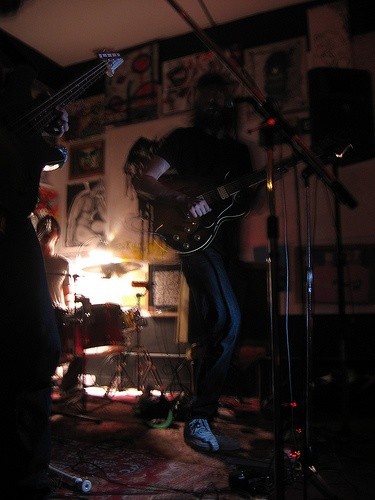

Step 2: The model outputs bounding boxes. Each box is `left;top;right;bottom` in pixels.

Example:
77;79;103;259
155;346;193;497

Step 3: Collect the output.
0;46;124;221
152;151;303;256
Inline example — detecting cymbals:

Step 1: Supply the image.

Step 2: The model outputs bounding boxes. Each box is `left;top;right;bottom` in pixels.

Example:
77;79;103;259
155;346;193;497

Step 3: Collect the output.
81;261;141;276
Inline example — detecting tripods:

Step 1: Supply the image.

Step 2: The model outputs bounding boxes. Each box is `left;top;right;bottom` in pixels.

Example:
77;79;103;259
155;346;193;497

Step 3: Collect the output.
104;295;163;396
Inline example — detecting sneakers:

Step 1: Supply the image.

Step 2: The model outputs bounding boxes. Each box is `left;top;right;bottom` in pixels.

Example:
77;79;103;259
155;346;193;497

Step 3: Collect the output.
211;427;241;450
184;418;219;451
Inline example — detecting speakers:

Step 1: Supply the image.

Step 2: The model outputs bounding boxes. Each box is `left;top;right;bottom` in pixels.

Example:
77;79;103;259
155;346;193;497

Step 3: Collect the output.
148;263;182;311
306;65;374;168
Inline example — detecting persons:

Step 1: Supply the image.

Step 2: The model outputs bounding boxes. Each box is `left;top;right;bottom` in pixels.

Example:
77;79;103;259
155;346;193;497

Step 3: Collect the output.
122;71;291;453
66;179;110;247
0;50;76;500
70;142;103;178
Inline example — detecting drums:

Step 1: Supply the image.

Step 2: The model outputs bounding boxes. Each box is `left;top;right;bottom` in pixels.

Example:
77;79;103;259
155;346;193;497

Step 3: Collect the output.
69;303;126;355
54;308;76;365
121;310;145;332
